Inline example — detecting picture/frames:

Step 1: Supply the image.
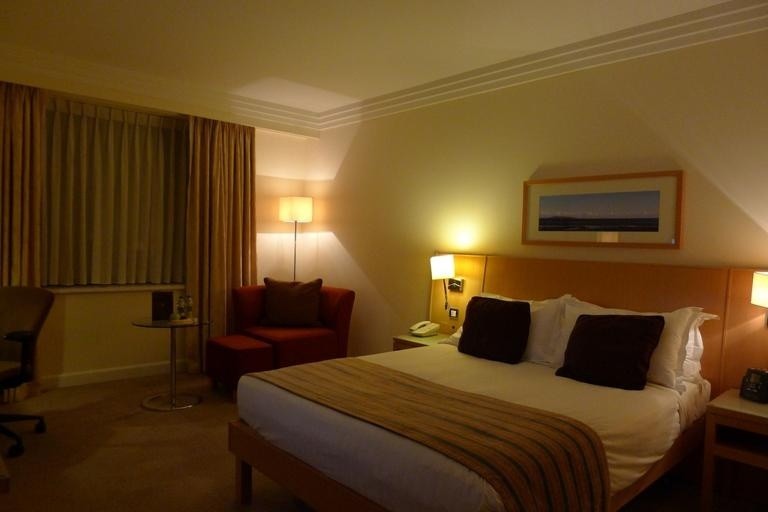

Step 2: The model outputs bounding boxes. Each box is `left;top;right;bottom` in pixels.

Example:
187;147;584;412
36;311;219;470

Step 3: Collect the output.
521;170;685;249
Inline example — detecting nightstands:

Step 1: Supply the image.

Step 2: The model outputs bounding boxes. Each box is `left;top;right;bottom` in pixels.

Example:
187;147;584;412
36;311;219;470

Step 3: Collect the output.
393;333;450;352
701;389;768;511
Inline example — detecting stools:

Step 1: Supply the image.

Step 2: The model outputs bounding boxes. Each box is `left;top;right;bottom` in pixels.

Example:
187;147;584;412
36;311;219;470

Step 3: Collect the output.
204;334;273;399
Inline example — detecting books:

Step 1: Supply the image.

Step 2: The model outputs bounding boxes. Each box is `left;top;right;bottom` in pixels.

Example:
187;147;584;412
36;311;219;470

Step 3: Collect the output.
172;317;193;325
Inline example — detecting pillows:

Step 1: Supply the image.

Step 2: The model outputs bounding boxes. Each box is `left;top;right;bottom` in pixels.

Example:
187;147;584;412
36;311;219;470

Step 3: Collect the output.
458;296;531;364
263;275;322;328
684;311;721;387
560;297;697;394
436;293;566;370
556;314;665;391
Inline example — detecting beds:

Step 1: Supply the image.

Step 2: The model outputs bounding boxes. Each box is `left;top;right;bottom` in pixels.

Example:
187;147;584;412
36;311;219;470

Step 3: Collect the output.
228;253;733;511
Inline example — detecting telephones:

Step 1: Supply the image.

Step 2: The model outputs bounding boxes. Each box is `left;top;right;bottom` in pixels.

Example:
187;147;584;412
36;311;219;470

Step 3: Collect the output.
409;320;440;337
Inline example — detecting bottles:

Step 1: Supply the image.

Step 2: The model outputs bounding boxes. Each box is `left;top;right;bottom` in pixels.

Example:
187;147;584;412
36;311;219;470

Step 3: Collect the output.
177;295;193;320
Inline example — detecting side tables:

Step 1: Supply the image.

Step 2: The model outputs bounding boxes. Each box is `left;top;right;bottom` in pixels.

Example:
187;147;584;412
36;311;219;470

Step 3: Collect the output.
131;317;211;410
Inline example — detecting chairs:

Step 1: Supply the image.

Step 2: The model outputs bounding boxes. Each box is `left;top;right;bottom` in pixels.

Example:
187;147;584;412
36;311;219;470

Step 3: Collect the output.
0;285;54;459
231;285;355;367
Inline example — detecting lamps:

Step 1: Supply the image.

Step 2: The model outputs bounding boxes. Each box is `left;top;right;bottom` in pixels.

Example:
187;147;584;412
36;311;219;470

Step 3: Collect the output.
750;271;768;326
279;195;313;281
429;254;464;311
